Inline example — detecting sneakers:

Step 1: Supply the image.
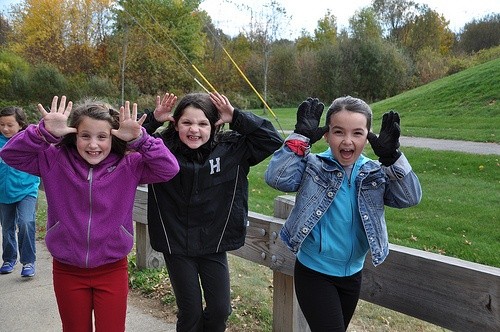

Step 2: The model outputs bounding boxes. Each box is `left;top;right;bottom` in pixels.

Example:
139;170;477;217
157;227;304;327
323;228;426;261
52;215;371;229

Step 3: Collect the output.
0;259;18;273
21;262;35;277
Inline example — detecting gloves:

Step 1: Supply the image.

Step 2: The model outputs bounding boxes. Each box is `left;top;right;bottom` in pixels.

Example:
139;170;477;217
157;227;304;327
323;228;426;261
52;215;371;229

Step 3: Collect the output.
294;97;329;145
367;111;402;167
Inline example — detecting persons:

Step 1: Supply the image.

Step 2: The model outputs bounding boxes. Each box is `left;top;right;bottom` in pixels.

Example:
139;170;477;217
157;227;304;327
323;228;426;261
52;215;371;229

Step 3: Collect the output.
0;105;42;278
0;95;181;332
133;93;284;332
264;96;422;332
